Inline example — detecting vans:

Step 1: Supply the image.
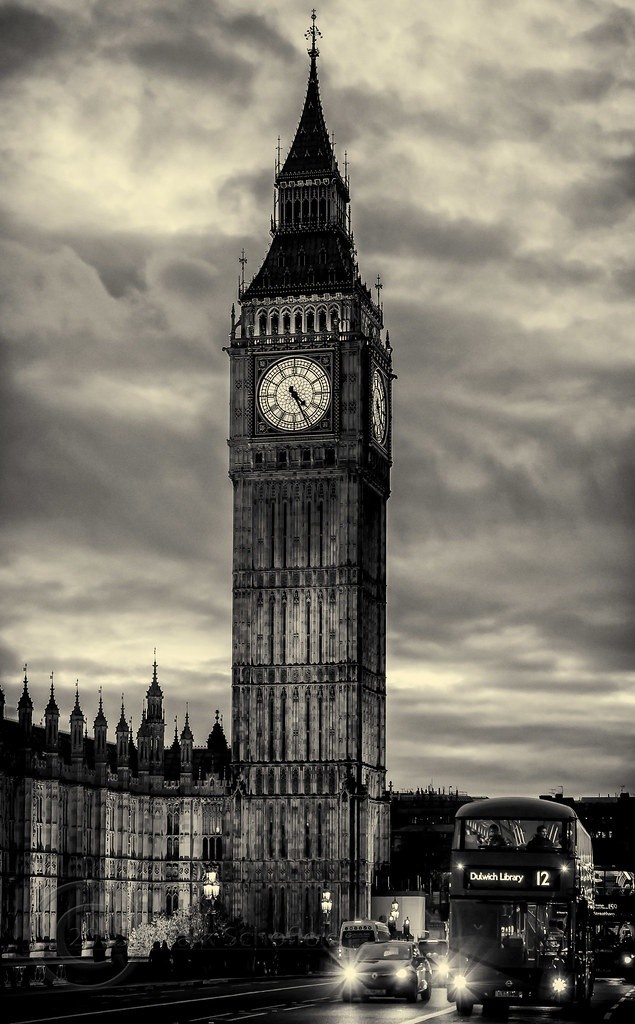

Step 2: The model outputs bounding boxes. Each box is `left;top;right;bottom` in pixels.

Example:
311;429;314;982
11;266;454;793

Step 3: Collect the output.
338;920;391;962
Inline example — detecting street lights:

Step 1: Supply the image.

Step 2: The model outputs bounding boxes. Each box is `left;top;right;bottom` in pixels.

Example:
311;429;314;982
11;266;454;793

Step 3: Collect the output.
203;870;220;933
321;892;333;936
390;898;399;922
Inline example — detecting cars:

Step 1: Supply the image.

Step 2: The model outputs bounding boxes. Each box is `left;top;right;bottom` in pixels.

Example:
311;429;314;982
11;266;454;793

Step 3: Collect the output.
343;941;432;1003
417;939;447;989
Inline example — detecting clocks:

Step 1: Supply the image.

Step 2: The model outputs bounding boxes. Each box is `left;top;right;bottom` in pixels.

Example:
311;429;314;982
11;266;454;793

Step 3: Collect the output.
370;367;389;449
256;355;334;435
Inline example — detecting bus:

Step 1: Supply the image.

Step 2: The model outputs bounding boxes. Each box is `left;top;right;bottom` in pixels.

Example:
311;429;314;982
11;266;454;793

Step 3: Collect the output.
446;797;596;1017
594;865;635;980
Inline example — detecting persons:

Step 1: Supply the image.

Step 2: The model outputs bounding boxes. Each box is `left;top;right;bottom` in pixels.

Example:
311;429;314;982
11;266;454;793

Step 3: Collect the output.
519;824;564;848
483;824;507;847
149;933;330;976
592;874;633;895
395;946;409;960
93;935;129;984
